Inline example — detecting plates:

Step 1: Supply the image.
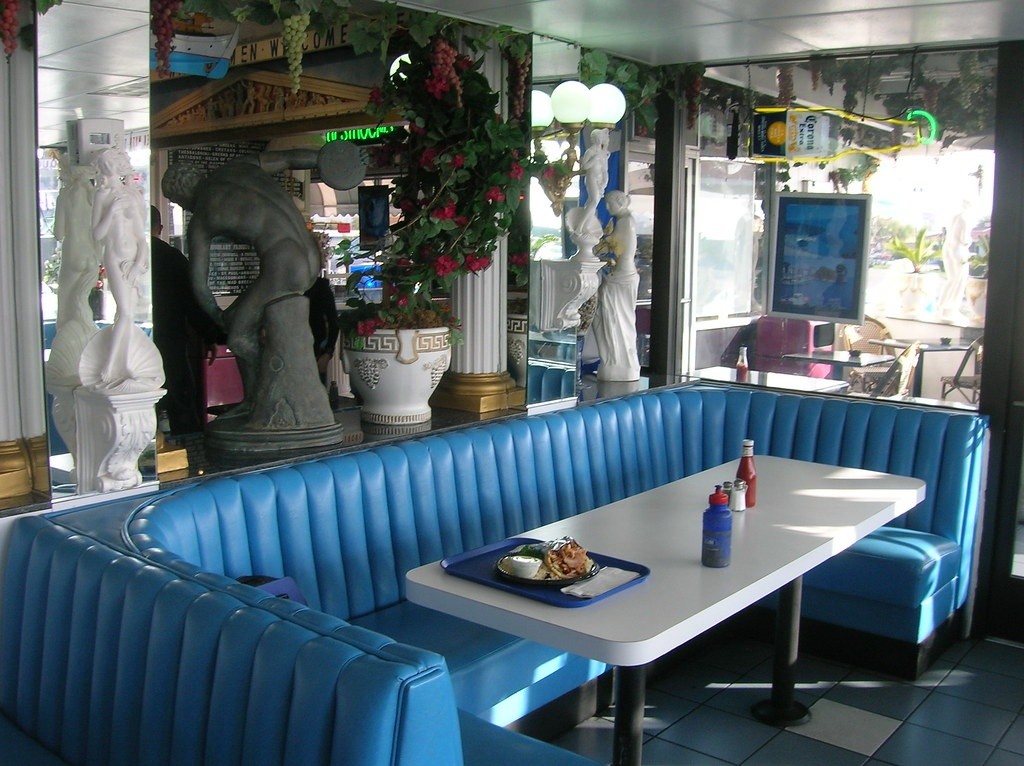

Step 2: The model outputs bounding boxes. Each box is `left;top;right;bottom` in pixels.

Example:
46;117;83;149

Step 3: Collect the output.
495;553;601;589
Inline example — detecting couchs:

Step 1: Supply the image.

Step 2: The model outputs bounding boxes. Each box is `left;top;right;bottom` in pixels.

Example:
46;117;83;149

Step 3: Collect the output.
0;382;990;765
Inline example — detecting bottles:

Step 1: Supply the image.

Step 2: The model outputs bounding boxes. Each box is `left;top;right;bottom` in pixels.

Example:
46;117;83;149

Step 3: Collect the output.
735;439;758;508
699;484;733;567
722;478;748;512
735;346;751;385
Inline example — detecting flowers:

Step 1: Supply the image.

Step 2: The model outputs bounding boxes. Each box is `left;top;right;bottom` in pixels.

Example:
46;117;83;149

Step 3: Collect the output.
591;218;623;276
334;35;570;348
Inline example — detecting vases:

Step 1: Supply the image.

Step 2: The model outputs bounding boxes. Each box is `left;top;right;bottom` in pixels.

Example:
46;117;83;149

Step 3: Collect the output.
342;326;451;426
576;292;598;337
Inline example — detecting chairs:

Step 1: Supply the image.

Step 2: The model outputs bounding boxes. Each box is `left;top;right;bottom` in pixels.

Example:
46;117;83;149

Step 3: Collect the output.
871;340;924;399
753;316;831;380
842;317;902;396
940;335;983;405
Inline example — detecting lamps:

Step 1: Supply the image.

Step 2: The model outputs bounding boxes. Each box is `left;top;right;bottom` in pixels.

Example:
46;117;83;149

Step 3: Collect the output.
531;90;561;217
549;80;625;217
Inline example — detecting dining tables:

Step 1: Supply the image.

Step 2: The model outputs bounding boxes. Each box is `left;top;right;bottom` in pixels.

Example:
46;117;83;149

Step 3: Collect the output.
403;455;926;766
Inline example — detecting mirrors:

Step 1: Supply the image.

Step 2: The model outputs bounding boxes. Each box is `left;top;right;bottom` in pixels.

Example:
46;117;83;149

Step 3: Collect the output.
522;30;581;411
31;0;157;505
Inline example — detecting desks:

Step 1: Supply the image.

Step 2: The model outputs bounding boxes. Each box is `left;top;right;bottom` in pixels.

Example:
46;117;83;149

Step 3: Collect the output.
694;366;848;394
781;350;896;380
867;336;977;399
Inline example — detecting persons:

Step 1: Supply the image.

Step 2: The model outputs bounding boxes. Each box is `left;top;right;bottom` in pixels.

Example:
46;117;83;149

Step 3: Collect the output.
162;163;320;418
574;129;609;233
91;147;149;383
591;190;641;364
735;204;762;297
942;212;972;305
304;278;340;387
561;135;577;172
151;205;218;437
54;153;99;330
534;138;545;155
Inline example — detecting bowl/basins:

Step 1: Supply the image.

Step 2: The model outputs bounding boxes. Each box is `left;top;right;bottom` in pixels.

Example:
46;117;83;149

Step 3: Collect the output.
940;337;952;345
848;349;862;357
509;554;543;579
